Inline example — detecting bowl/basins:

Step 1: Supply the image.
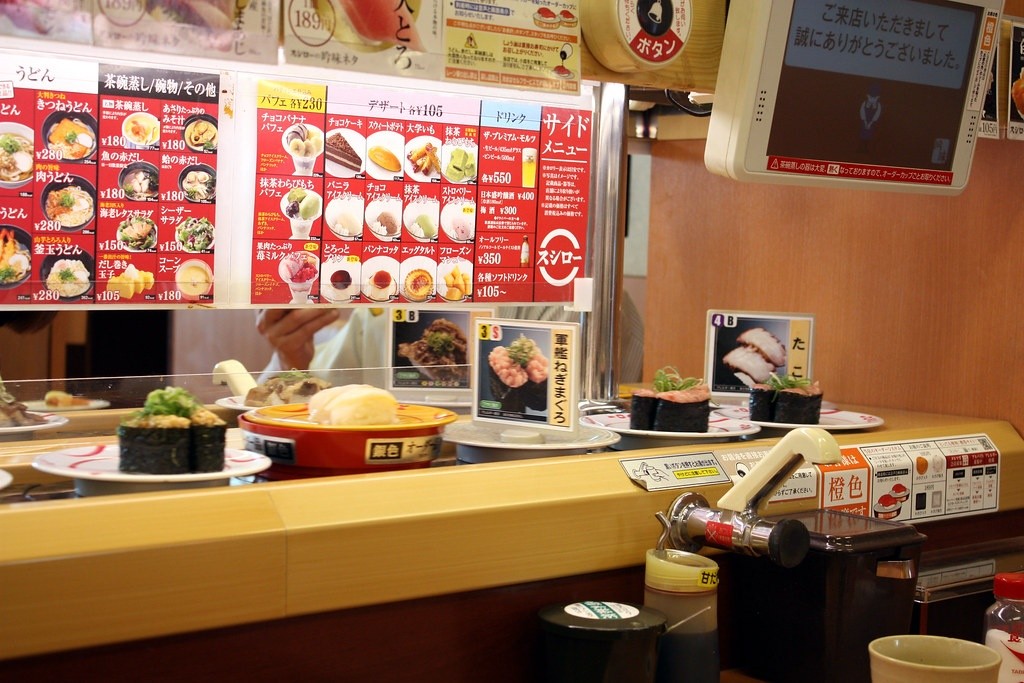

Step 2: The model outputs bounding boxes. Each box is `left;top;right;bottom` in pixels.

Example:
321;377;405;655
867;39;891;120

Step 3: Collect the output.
118;161;160;202
181;114;218;153
178;163;216;203
1;108;98;301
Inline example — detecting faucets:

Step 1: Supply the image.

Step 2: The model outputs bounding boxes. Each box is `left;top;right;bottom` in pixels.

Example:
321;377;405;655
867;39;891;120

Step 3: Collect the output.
665;425;842;569
209;358;256;398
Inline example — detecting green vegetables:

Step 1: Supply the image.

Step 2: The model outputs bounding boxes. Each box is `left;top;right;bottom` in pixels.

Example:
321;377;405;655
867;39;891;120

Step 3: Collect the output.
506;334;538;367
64;130;79;145
0;134;20;153
0;266;17;281
59;267;76;283
765;371;813;403
129;385;204;418
651;365;702;393
59;193;75;207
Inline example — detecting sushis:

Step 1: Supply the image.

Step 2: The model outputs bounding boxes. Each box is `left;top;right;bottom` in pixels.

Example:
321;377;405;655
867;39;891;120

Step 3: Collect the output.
628;389;712;433
748;384;824;425
116;411;227;475
309;383;399;427
723;327;787;383
489;346;551;414
399;318;467;383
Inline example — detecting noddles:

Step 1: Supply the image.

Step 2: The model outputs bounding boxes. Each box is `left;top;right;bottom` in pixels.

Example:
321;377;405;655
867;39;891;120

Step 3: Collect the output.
45;186;94;227
47;118;96;160
0;133;34;182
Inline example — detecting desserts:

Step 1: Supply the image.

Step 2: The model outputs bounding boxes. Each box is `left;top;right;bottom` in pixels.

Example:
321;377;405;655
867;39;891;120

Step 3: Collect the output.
279;124;476;301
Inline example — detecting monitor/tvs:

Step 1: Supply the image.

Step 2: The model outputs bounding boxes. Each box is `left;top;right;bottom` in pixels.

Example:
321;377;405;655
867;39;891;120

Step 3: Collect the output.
703;0;1005;196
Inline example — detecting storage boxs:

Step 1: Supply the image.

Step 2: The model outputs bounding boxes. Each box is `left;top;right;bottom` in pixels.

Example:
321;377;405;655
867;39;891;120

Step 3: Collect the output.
724;508;928;683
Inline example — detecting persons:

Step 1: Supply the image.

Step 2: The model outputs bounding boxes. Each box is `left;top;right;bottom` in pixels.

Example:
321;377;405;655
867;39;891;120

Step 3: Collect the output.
252;308;387;387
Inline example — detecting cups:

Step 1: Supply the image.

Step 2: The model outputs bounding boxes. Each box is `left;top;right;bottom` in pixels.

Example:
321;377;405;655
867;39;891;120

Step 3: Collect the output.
278;251;320;303
282;124;325;177
868;634;1003;683
280;188;323;240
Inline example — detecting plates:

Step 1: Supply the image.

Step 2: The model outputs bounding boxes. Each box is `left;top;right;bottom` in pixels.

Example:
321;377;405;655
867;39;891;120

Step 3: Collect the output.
0;468;13;490
321;128;479;303
579;413;761;440
32;445;273;483
19;399;110;412
443;420;621;450
710;408;884;429
0;411;69;435
215;396;259;411
116;219;216;254
122;111;160;146
243;403;459;431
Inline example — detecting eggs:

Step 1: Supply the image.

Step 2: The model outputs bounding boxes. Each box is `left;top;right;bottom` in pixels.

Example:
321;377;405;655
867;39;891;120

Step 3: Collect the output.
187;171;210;183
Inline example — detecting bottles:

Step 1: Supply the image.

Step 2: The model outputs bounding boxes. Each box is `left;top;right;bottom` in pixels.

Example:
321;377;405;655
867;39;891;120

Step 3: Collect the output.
983;572;1024;683
